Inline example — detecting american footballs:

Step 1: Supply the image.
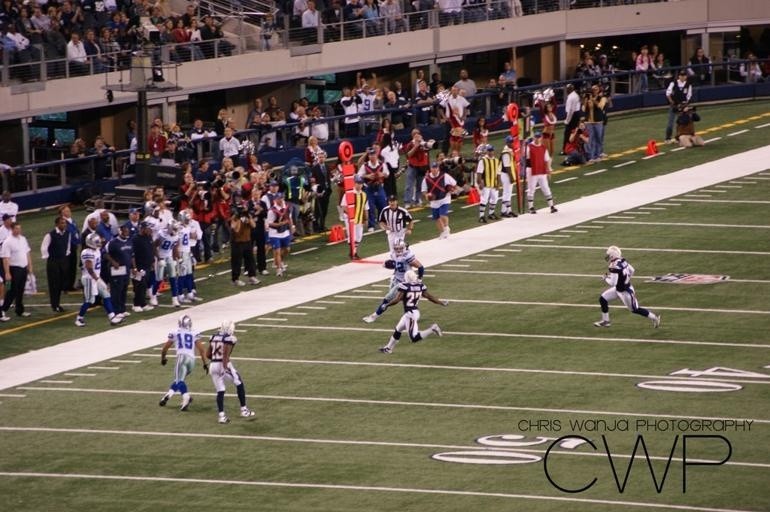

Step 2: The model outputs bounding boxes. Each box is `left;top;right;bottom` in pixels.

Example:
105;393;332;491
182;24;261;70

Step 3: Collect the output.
385;260;396;268
426;193;433;200
446;185;455;191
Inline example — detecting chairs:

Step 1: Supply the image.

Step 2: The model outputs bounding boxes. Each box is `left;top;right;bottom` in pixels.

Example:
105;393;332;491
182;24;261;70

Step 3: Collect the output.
27;0;344;75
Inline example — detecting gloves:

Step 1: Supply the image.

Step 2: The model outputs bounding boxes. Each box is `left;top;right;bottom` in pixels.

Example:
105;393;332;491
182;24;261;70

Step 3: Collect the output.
381;304;387;310
442;301;449;306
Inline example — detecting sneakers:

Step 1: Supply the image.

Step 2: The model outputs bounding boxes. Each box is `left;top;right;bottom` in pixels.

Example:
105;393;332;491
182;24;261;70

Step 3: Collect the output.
600;152;607;158
362;315;375;323
527;207;535;213
160;394;171;406
240;409;255;417
111;317;124;325
431;323;442;337
443;226;451;238
440;231;447;240
180;396;193;411
550;206;557;213
378;347;393;355
1;299;31;322
479;211;517;223
594;320;610;327
219;417;231;423
231;262;288;287
75;319;85;327
654;315;660;328
116;286;197;319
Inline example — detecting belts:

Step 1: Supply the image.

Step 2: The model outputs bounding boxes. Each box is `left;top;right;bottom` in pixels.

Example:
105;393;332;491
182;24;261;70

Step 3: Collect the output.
211;359;223;362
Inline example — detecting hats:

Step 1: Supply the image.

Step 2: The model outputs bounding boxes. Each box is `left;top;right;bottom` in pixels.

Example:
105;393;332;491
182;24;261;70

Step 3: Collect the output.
129;208;142;214
2;214;13;220
432;162;437;167
534;132;541;138
506;136;513;142
487;145;494;150
354;174;364;183
679;70;686;75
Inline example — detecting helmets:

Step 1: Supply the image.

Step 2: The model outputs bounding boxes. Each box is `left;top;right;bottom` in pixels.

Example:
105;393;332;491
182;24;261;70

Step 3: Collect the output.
394;238;406;256
221;321;234;335
178;315;192;329
85;233;103;250
605;246;621;262
144;200;191;236
404;270;416;283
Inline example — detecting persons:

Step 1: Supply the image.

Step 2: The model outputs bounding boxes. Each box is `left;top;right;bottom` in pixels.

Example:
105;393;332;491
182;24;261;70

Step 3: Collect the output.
160;315;208;410
205;319;255;423
380;271;451;354
